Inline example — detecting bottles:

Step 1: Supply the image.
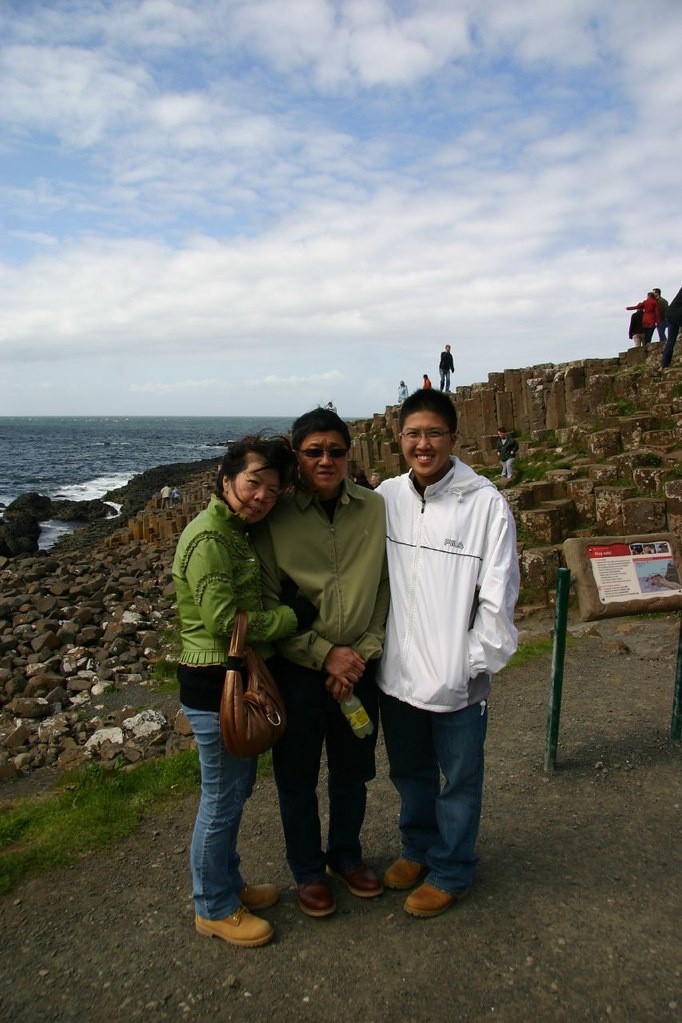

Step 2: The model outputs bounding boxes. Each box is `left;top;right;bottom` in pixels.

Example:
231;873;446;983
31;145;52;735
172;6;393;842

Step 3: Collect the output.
339;691;374;739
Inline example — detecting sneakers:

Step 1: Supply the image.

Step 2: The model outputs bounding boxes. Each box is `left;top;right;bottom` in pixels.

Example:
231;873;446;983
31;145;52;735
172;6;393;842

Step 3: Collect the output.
197;904;273;947
326;861;383;896
296;883;336;917
384;859;425;890
404;883;470;918
238;882;280;910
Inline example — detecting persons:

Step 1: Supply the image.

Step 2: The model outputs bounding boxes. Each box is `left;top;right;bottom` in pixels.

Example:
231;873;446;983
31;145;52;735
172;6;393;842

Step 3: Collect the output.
373;388;521;918
160;483;171;510
439;345;454;393
172;428;318;948
497;427;519;479
328;402;337;414
626;287;682;369
398;380;408;404
423;374;431;389
265;406;390;920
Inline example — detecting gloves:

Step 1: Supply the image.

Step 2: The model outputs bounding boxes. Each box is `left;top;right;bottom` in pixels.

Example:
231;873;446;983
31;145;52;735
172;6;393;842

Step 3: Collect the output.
281;581;317;632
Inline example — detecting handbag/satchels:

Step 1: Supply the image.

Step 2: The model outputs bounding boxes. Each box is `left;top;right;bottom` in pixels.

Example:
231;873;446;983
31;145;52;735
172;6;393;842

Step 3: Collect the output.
219;611;286;760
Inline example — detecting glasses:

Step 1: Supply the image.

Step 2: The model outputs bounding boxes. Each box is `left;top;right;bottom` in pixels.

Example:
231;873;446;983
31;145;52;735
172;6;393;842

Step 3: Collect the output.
301;448;348;458
399;429;450;442
